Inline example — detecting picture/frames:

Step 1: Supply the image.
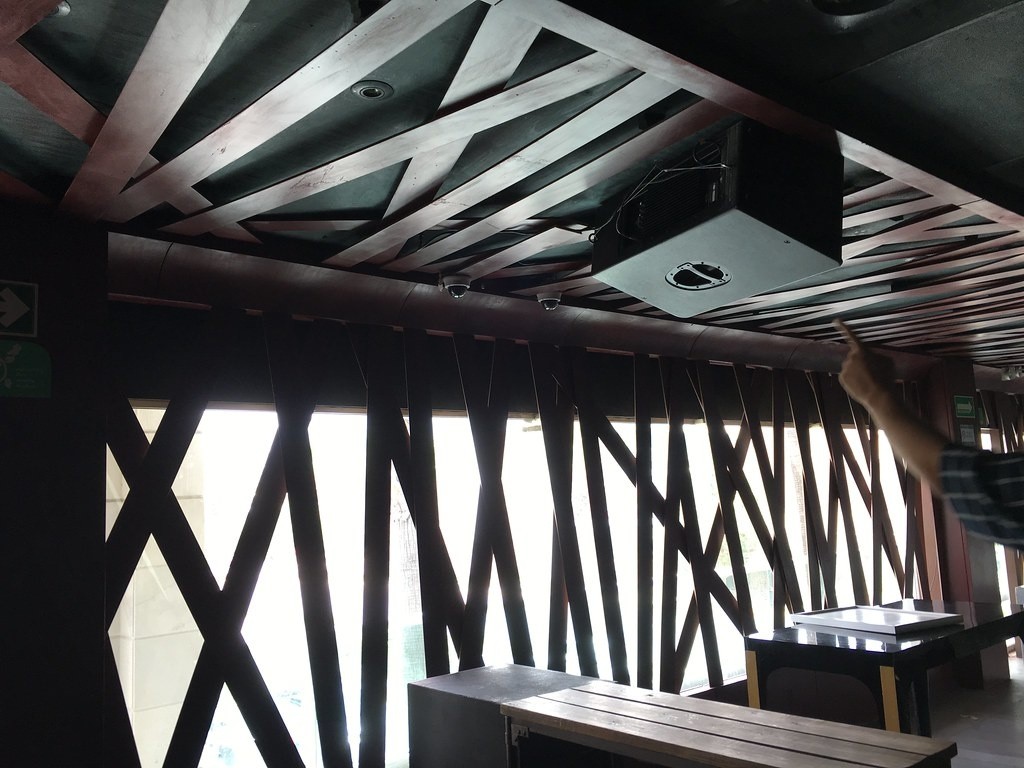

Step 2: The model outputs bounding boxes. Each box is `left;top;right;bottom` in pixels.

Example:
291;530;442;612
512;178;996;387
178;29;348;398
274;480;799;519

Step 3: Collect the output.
791;605;964;635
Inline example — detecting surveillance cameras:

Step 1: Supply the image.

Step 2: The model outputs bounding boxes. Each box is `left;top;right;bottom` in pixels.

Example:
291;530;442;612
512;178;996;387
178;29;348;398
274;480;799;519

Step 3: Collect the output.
537;291;563;310
442;275;472;298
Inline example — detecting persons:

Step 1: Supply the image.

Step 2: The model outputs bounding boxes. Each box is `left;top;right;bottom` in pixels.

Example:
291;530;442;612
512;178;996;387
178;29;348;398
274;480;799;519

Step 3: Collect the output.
837;317;1024;549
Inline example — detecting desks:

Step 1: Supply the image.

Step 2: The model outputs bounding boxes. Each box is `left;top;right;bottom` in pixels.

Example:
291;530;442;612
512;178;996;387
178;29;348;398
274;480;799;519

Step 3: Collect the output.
744;597;1024;732
499;678;958;768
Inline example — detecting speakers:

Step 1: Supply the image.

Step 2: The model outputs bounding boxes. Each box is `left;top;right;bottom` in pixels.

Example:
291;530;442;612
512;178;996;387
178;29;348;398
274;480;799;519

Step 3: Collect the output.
591;116;844;319
